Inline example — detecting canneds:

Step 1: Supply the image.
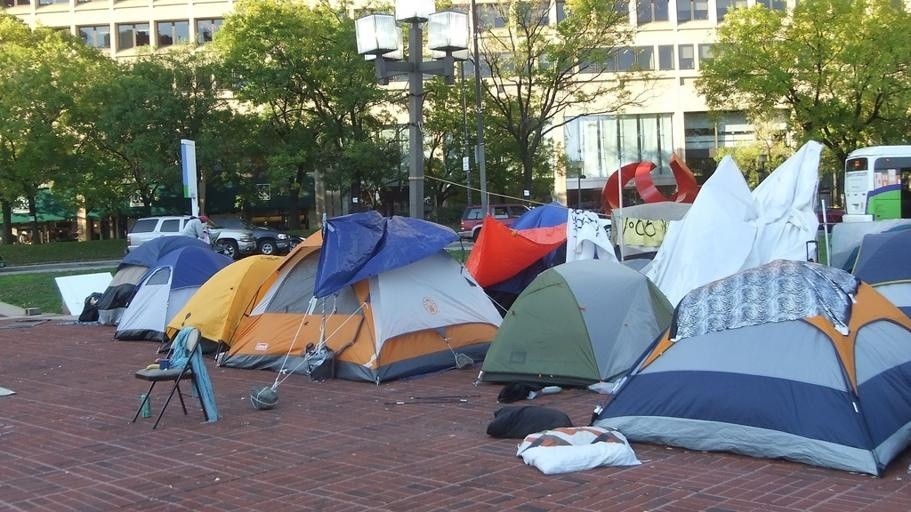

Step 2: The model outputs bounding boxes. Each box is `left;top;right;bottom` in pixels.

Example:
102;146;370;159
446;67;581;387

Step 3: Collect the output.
140;393;151;418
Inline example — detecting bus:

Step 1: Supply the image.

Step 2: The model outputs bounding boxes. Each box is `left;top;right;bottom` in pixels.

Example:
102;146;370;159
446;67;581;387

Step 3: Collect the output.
842;145;911;222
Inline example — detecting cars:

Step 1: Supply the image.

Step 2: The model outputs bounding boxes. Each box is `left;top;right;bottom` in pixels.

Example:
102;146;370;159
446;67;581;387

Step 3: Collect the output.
126;215;307;260
458;204;532;243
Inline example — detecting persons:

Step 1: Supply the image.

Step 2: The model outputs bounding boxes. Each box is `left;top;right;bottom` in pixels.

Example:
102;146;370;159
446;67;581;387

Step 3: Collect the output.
19;230;33;244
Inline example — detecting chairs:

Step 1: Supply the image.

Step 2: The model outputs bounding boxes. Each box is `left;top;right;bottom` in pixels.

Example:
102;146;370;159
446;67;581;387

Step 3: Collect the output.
131;326;207;430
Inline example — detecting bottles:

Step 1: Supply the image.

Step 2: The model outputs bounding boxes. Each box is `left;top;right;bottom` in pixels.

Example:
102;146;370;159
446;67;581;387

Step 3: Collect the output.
142;392;152;420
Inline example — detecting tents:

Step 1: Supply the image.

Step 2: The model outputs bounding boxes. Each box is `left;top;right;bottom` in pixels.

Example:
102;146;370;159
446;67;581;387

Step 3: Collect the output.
96;145;911;477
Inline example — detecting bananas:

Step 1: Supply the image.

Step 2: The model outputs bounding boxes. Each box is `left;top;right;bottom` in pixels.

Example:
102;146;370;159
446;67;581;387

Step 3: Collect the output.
146;364;160;370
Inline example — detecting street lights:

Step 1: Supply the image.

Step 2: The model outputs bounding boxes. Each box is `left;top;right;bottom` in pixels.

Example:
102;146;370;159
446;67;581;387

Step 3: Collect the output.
577;167;586;208
355;0;469;220
